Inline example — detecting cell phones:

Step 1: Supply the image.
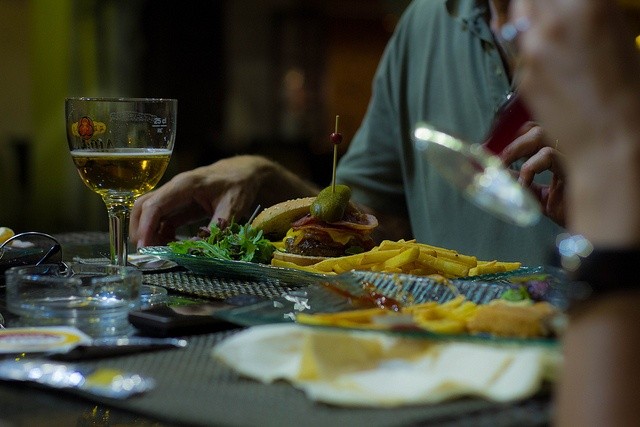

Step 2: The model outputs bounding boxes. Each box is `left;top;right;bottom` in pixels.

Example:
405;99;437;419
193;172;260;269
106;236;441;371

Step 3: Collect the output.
127;295;264;339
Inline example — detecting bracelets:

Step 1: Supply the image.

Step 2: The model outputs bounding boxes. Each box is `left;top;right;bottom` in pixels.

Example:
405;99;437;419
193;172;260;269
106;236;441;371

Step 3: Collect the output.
551;248;640;294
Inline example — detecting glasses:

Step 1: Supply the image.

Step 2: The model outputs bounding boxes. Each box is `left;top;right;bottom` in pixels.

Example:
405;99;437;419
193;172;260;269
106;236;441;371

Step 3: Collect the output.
0;233;62;290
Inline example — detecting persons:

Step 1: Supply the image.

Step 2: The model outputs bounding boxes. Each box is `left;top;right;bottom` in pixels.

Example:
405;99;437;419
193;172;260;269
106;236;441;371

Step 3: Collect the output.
515;1;640;427
128;0;571;267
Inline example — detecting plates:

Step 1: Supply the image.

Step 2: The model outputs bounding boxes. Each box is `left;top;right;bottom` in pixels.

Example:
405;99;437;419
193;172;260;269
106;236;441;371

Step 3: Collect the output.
169;248;336;284
212;268;559;349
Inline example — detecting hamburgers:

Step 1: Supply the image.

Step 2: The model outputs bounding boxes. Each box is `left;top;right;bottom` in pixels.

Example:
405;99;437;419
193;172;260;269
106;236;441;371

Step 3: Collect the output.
250;182;384;266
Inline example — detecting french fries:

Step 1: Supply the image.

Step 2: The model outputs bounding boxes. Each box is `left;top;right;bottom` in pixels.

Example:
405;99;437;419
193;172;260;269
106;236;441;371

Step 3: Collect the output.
289;294;497;333
286;237;528;285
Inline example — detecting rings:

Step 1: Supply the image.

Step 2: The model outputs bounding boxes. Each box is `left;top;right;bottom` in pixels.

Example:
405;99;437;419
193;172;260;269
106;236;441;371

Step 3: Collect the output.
555;138;559;151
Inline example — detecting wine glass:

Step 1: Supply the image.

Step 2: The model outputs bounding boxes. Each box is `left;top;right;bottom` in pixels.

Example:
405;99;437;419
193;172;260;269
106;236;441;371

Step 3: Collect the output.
62;95;180;302
410;86;545;230
